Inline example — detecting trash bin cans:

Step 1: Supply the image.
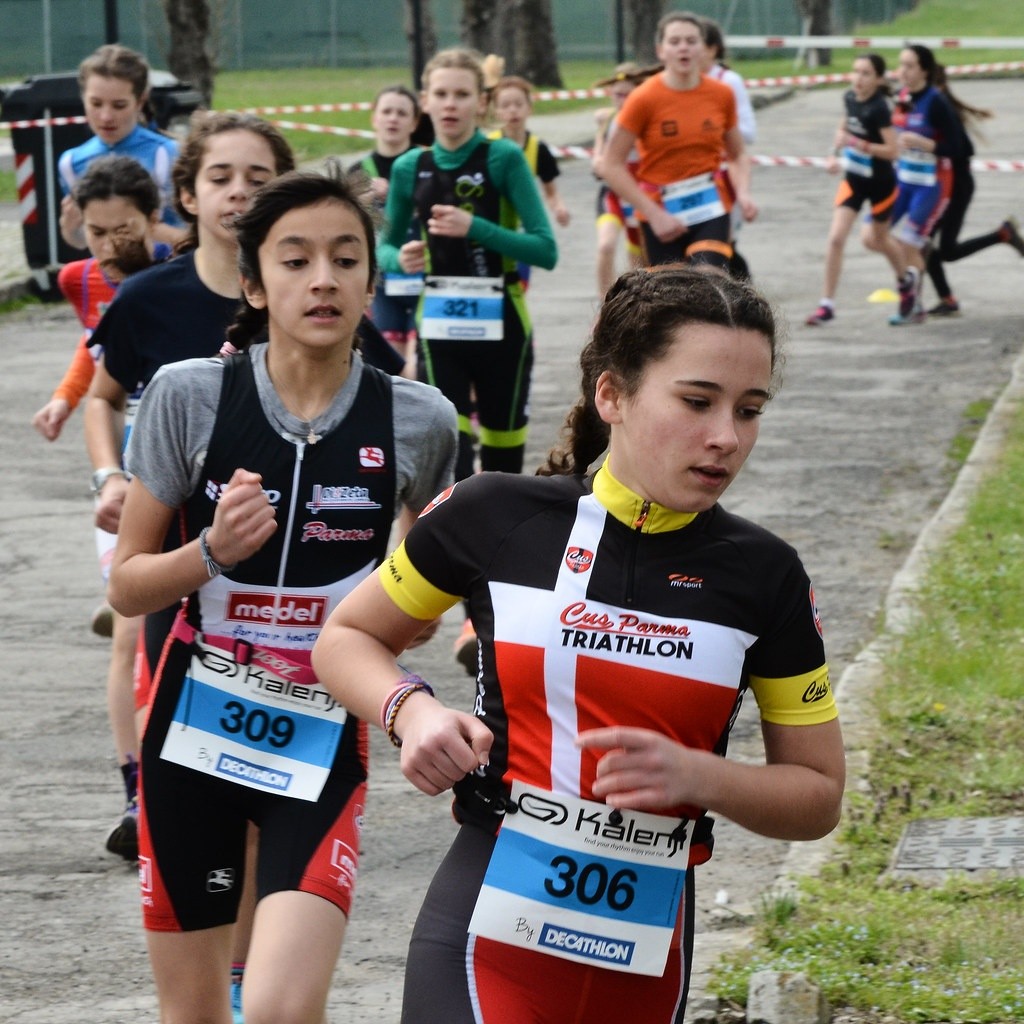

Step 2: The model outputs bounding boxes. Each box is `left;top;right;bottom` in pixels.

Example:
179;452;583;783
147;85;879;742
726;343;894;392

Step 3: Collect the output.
0;69;204;303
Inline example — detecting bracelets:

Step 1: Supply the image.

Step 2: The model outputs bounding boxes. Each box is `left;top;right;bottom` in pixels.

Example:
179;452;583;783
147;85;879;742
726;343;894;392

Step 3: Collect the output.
380;674;434;748
199;527;238;579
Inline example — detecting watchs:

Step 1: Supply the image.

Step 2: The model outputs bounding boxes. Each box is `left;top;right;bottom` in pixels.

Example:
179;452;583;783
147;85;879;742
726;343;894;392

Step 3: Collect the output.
89;466;125;495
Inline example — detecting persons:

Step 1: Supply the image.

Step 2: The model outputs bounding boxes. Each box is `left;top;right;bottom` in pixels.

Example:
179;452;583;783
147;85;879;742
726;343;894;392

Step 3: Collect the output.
33;43;189;858
86;108;406;1024
805;46;1024;325
308;265;846;1024
345;45;573;666
104;172;457;1024
598;12;752;309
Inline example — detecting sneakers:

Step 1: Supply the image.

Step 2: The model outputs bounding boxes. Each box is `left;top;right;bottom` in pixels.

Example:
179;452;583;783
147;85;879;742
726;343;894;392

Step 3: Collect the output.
807;307;834;324
106;805;139;867
928;299;958;313
999;220;1024;256
898;266;919;318
